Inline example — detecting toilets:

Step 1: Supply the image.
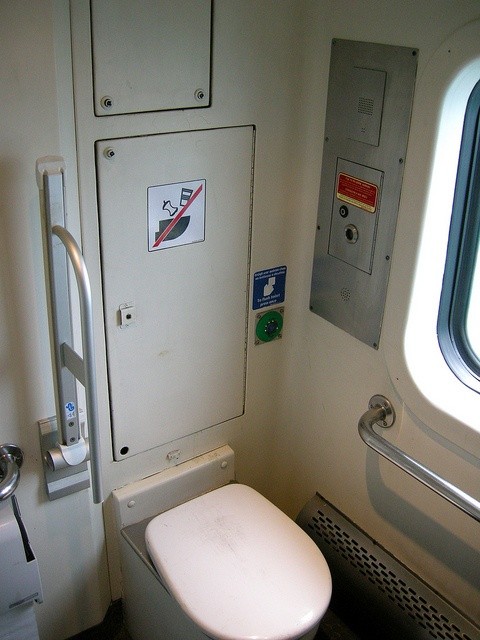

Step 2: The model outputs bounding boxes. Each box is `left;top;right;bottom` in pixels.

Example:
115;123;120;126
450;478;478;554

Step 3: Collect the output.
102;443;333;638
